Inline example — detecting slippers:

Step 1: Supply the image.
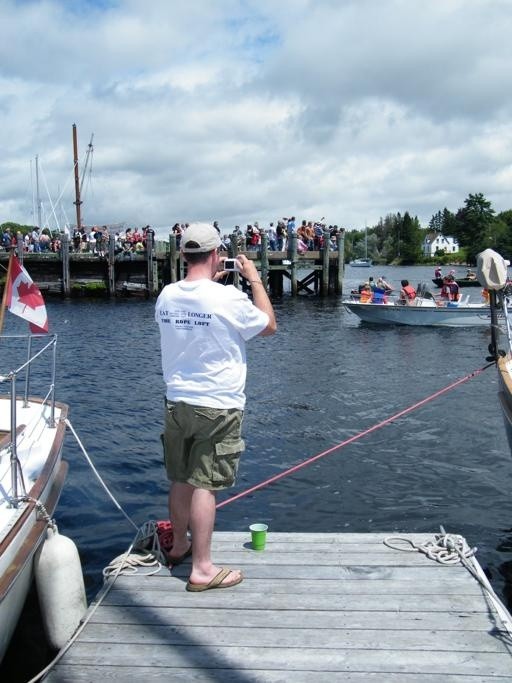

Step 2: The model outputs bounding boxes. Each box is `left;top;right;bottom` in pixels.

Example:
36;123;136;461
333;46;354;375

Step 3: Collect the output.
168;539;193;565
186;566;244;592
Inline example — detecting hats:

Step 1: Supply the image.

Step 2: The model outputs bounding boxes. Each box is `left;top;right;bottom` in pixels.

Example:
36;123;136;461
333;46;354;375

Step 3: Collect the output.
180;223;227;255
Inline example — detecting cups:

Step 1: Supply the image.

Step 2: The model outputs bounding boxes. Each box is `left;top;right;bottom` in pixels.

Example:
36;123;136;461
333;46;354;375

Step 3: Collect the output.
249;523;269;552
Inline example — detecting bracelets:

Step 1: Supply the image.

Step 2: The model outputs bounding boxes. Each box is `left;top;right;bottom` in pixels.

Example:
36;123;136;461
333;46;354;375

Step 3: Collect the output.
246;279;263;285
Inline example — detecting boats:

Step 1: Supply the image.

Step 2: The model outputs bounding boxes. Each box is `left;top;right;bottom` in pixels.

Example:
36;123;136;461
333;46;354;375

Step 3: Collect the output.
341;278;512;329
349;258;371;268
1;333;68;652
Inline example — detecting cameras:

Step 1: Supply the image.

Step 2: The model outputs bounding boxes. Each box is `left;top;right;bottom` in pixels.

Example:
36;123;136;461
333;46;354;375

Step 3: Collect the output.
224;259;240;272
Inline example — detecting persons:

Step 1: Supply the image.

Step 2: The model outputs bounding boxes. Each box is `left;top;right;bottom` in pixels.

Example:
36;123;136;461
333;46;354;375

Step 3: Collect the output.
172;222;245;255
155;221;277;591
350;266;477;306
245;216;339;255
0;225;155;255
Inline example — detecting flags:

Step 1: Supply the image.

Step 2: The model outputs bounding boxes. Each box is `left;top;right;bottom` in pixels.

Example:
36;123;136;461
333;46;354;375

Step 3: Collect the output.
6;253;49;333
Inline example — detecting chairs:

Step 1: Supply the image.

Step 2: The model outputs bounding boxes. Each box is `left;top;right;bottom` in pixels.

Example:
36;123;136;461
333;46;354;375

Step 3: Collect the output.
454;294;463;304
464;294;470;306
